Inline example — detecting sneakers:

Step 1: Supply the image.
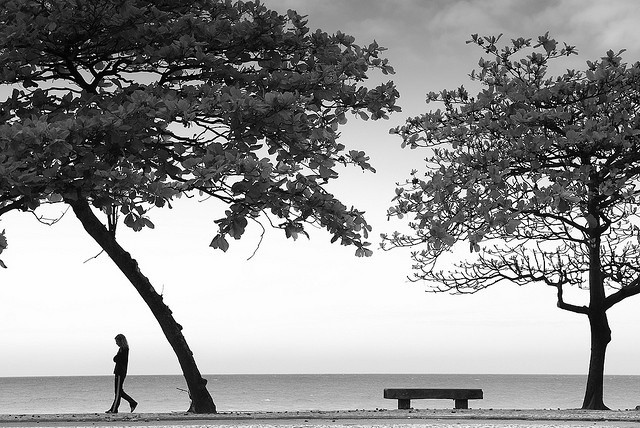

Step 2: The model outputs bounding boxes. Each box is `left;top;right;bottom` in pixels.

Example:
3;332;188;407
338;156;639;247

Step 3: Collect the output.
105;410;118;413
131;403;138;412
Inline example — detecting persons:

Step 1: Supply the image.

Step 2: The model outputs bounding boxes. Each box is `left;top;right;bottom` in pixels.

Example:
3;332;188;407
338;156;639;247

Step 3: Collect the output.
105;334;138;414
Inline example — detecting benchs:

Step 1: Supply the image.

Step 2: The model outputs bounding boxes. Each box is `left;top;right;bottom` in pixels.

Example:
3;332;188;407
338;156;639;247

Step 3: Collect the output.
384;389;483;409
